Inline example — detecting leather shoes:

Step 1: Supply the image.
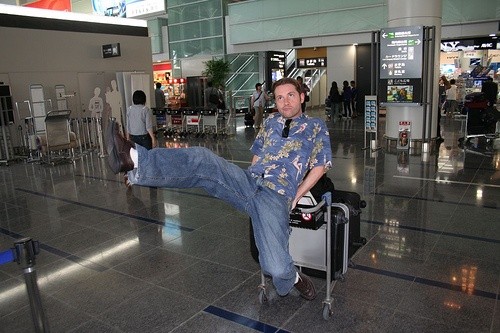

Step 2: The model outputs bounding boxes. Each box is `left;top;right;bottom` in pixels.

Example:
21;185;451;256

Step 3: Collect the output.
100;116;134;175
294;269;317;300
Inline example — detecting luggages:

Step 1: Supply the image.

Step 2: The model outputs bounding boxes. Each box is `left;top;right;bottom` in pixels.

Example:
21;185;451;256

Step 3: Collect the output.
248;202;357;282
245;108;254;121
334;189;368;258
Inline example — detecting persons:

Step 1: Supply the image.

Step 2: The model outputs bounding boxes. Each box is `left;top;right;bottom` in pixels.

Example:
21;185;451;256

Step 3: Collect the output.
89;88;103;147
342;81;351;118
127;90;155;151
297;76;310;113
350;81;357;117
155;82;165;109
105;80;124;138
252;83;270;129
439;76;445;95
446;79;457;111
203;79;227;115
330;82;340;120
107;78;331;300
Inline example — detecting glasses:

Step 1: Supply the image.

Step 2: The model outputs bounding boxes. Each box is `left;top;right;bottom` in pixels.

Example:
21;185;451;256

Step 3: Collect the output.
283;119;293;138
